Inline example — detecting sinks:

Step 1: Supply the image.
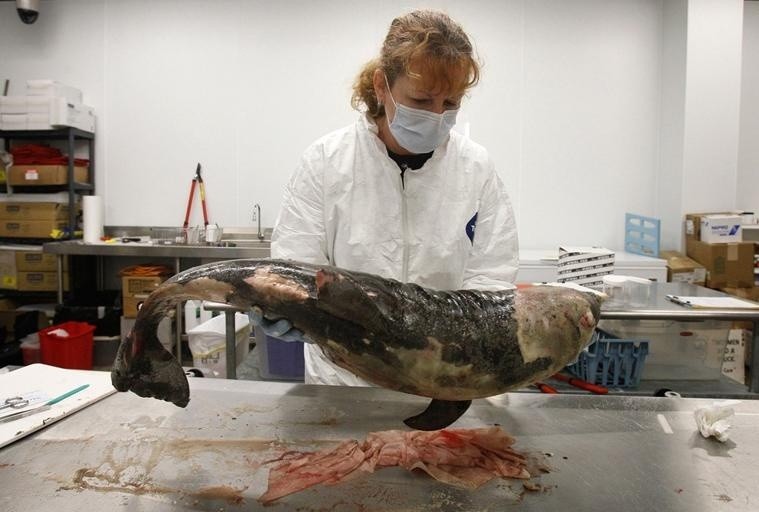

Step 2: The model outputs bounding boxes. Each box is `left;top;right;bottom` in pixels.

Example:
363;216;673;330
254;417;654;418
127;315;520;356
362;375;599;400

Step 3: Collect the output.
199;239;272;265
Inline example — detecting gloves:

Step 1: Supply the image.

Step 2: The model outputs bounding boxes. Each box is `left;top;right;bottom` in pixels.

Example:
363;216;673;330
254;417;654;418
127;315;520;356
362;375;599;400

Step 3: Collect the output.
248;305;316;345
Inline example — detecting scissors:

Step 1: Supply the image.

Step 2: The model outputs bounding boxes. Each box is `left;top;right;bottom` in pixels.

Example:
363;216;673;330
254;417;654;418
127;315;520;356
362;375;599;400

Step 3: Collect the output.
0;395;29;411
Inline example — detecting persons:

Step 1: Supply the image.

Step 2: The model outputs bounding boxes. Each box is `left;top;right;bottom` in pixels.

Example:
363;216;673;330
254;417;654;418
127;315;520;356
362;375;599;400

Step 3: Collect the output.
246;9;518;389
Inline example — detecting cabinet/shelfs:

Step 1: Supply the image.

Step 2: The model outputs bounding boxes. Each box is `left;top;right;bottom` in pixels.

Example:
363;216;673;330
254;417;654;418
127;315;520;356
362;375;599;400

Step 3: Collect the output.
0;116;96;332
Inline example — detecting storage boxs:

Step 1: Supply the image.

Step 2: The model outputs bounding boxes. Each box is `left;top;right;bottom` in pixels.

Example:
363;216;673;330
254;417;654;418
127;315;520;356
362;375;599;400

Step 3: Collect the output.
0;247;70;292
610;314;731;384
658;208;759;306
19;317;120;370
0;200;82;239
6;162;90;188
120;275;167;320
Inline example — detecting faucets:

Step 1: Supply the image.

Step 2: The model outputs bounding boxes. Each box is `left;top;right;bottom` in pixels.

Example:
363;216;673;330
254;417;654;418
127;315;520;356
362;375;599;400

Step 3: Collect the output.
251;204;264;240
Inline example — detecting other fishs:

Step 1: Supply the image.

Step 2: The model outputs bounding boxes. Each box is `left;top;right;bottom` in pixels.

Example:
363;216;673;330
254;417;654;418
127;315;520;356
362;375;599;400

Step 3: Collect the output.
110;257;605;431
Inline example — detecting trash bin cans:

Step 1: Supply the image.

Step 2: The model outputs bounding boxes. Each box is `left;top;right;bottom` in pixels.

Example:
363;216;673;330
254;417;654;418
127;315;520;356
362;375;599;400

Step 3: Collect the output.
40;319;97;368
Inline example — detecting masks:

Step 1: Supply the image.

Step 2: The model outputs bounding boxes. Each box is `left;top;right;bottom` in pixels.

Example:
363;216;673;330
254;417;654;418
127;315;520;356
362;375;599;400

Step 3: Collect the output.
383;71;461;154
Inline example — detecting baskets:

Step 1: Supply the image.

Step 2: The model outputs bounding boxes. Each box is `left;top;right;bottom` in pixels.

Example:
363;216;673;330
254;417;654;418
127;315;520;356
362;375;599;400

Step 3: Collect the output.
557;246;615;290
565;327;650;389
